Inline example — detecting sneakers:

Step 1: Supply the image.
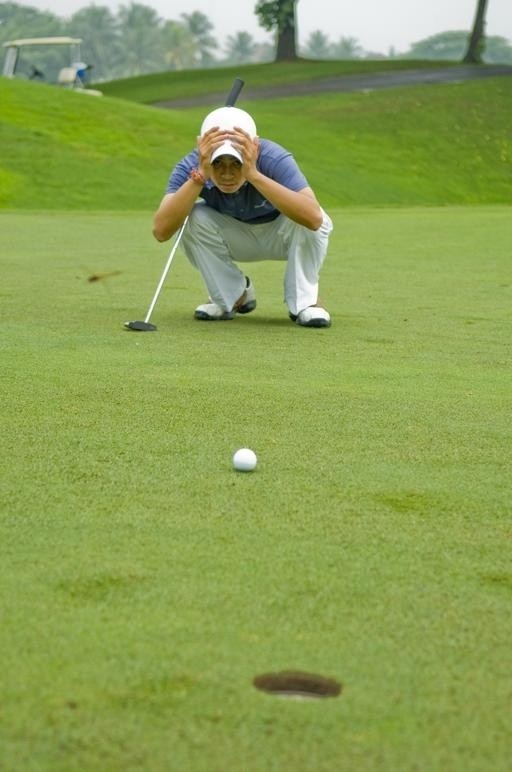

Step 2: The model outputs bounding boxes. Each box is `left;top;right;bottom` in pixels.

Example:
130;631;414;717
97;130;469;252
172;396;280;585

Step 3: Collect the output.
288;304;331;328
194;275;257;320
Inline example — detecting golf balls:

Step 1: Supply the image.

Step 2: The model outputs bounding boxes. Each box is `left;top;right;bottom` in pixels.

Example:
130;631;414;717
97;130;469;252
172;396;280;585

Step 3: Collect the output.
233;449;256;471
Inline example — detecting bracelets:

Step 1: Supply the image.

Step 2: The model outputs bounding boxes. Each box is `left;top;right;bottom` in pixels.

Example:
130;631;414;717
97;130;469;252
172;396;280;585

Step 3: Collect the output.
190;166;206;186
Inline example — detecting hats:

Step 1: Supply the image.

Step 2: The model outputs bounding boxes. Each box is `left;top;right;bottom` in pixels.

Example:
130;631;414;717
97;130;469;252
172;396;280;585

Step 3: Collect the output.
200;105;258;165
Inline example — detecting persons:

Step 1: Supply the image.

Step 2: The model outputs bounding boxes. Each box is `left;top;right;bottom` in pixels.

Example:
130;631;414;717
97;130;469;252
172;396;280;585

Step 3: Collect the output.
27;63;46;82
68;61;95;89
153;104;334;328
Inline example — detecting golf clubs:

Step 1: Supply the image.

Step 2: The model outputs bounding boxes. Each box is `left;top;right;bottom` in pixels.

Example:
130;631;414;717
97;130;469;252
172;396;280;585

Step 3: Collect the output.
123;80;246;331
